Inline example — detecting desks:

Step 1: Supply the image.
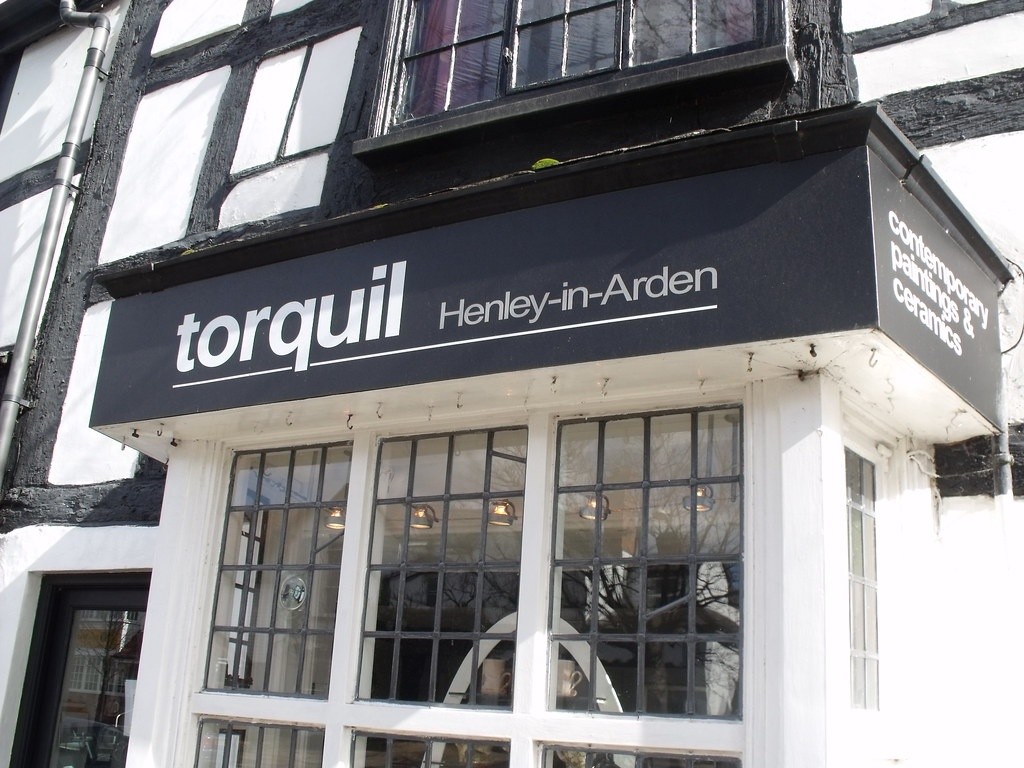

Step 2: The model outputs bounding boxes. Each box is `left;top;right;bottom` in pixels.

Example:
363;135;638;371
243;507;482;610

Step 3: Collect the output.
450;690;606;768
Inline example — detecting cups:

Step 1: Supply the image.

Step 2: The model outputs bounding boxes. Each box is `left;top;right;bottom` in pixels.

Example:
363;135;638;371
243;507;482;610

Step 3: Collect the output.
558;660;582;697
481;659;511;695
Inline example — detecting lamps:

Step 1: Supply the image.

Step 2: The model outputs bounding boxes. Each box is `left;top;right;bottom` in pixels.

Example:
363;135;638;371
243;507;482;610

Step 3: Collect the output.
410;503;440;529
326;506;345;529
579;493;611;522
683;484;716;512
487;497;517;526
